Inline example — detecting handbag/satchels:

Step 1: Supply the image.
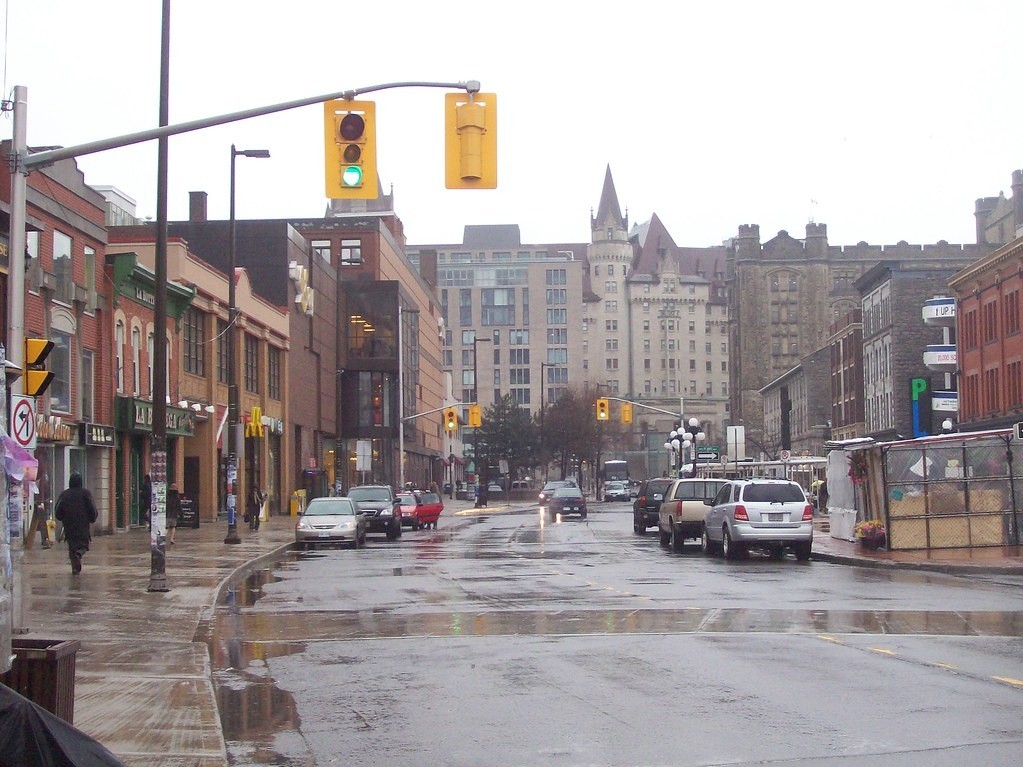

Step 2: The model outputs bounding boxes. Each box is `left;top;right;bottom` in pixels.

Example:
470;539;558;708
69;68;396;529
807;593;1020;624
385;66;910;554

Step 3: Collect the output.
242;514;250;522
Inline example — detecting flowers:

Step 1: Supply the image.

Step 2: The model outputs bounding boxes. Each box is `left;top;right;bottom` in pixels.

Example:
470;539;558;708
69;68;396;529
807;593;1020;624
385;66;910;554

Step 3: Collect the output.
844;450;871;486
850;519;884;541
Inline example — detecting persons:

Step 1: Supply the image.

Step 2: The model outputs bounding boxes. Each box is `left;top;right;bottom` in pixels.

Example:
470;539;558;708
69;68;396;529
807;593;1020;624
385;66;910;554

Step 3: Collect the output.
329;484;336;497
430;482;438;493
246;485;264;530
166;484;184;545
54;472;98;575
141;475;151;532
419;488;437;530
411;481;415;492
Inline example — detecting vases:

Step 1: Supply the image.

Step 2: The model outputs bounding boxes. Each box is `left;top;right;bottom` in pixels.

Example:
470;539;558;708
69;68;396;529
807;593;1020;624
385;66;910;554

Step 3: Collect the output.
860;538;883;551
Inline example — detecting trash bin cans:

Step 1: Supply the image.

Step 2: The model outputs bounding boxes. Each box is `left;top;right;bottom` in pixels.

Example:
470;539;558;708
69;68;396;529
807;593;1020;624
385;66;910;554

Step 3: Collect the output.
12;638;82;729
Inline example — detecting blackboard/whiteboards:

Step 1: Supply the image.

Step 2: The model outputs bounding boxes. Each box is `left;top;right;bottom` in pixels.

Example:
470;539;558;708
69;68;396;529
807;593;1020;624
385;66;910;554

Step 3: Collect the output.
177;493;200;526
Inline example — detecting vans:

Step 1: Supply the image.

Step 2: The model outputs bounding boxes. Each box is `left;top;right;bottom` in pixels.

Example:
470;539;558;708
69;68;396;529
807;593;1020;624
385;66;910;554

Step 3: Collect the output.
511;480;529;490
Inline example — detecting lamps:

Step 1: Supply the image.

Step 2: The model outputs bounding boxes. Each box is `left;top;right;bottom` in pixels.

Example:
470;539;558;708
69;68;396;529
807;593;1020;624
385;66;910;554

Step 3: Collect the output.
137;395;171;407
171;401;188;408
183;399;201;411
198;402;215;413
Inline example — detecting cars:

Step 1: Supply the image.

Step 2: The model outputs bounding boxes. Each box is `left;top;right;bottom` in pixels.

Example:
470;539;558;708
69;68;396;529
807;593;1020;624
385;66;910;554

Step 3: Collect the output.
605;483;632;502
538;480;582;506
295;496;369;550
659;479;733;554
394;487;444;530
549;488;587;523
444;483;502;502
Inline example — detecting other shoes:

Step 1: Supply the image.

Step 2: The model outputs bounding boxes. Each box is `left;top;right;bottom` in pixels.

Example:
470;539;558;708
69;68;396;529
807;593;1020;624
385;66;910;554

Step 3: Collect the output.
169;541;175;544
74;554;82;571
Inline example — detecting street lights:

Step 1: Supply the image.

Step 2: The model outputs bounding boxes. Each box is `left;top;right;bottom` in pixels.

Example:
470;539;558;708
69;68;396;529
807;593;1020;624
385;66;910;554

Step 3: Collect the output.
664;417;706;479
540;362;556;489
224;149;271;544
474;337;492;507
570;453;580;477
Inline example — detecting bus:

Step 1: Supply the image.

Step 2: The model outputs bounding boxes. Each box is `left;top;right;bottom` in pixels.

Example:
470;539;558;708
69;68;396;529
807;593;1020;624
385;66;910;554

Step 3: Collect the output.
605;460;630;481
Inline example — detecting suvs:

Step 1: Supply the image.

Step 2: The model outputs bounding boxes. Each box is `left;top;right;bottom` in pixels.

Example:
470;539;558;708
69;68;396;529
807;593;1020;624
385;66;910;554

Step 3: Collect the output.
347;482;403;541
701;476;814;560
633;478;679;535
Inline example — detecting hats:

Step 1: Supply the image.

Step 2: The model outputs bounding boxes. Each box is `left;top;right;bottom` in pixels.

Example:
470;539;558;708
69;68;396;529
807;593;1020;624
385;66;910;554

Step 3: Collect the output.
169;483;179;490
250;482;260;490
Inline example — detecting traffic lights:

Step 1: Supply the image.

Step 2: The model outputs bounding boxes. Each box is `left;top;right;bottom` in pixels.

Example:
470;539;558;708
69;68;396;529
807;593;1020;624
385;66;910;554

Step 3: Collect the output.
22;337;57;396
445;408;458;430
324;100;378;199
597;399;609;421
